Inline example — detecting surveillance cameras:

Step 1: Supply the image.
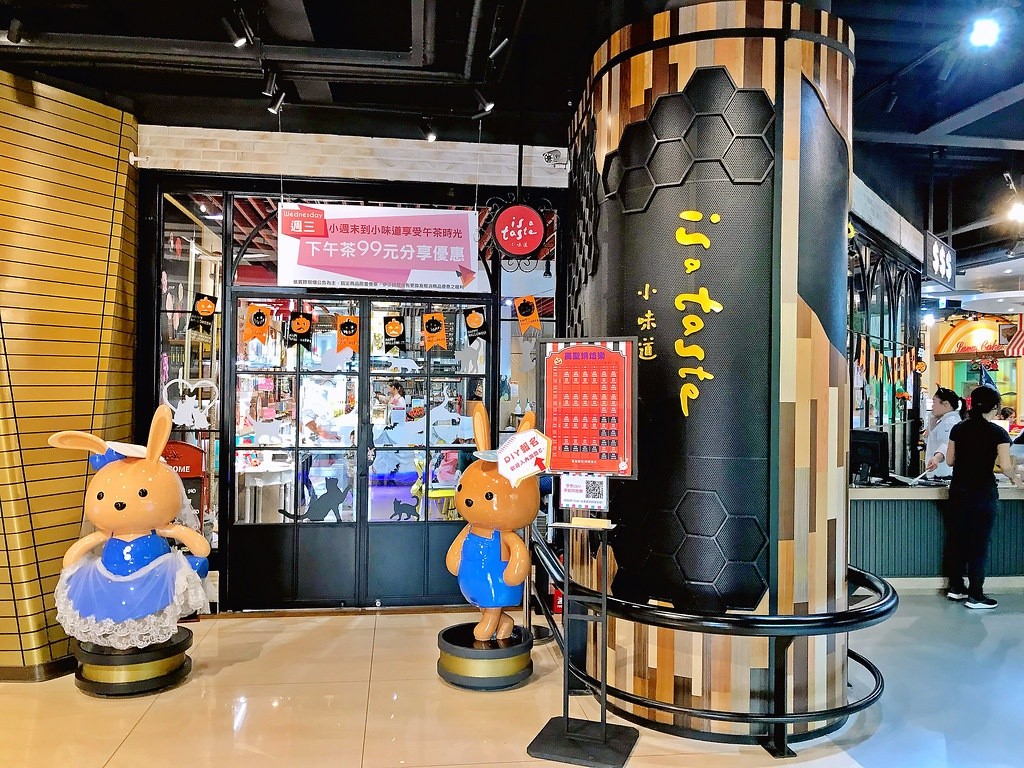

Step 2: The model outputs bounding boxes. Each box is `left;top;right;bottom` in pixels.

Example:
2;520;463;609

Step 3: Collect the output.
542;150;562;163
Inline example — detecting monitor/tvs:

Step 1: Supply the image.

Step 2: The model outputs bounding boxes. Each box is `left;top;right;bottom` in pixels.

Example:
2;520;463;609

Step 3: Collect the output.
850;430;889;488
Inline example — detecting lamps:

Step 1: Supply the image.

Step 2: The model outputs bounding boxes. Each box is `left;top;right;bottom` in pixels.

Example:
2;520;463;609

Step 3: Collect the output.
486;33;509;60
883;85;898;114
417;115;436;143
473;87;494;112
261;64;276;96
267;91;285;114
222;13;246;47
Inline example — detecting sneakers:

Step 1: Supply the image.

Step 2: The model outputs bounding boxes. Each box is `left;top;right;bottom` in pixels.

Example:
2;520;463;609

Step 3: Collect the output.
946;588;969;600
965;594;999;609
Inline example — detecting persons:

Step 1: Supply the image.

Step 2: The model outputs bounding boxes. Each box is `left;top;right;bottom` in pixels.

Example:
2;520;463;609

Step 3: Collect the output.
999;408;1016;424
300;376;341;446
373;379;406;428
946;387;1021;609
924;388;967;479
